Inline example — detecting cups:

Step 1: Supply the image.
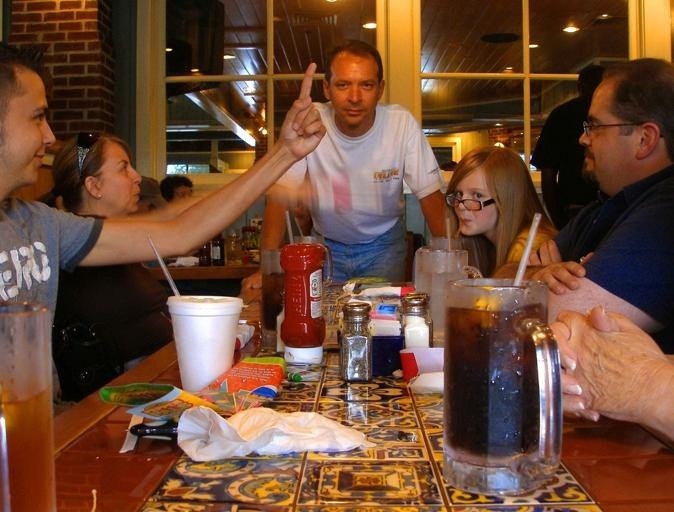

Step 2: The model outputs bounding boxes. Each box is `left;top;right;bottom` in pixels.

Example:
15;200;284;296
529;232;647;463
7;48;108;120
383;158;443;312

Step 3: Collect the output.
0;298;61;512
444;280;565;499
259;246;286;347
414;248;484;347
166;293;244;396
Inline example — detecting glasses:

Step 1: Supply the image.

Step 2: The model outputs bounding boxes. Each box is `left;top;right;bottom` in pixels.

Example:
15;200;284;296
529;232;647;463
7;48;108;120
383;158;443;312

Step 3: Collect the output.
582;120;665;137
445;192;495;211
77;132;100;187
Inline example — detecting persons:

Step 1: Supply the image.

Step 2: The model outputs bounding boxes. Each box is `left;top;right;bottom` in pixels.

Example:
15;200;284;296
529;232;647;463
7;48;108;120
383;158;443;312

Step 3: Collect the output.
240;39;452;332
548;304;674;448
0;39;326;406
38;131;174;405
528;63;606;229
494;56;674;335
132;178;169;216
446;144;559;285
159;173;194;202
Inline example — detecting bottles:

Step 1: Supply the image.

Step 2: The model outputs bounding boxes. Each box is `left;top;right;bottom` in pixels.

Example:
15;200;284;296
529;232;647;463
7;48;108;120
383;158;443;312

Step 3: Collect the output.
279;242;328;370
339;302;374;384
402;292;434;349
198;231;226;267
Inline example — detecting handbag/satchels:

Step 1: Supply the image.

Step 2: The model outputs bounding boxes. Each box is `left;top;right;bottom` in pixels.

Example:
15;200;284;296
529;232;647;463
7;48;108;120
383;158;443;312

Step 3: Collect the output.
54;308;126;403
